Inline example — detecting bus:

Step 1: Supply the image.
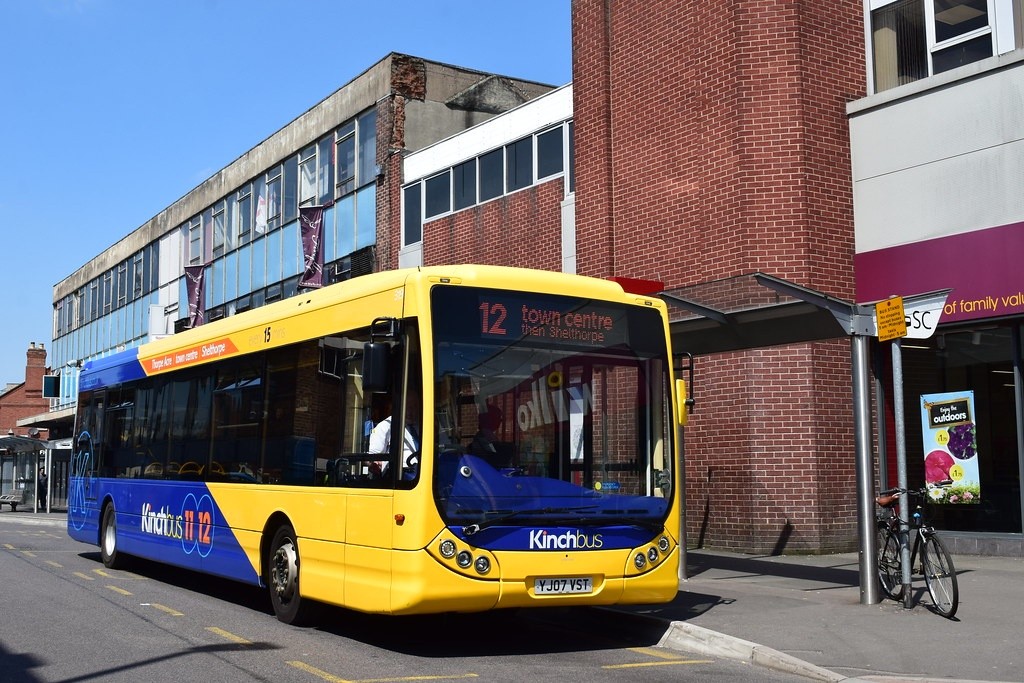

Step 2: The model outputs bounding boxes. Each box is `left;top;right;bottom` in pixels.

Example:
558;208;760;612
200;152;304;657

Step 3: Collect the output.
67;264;696;624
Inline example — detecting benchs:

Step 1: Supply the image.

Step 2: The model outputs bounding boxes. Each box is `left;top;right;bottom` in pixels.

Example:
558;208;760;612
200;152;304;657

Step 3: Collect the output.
0;489;29;511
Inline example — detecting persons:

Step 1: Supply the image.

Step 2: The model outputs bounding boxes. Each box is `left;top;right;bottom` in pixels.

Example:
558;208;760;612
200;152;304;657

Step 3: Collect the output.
369;391;451;472
468;403;503;450
39;466;48;509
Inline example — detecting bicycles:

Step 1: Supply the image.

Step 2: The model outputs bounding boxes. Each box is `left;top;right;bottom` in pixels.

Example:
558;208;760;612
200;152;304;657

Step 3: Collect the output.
875;478;959;621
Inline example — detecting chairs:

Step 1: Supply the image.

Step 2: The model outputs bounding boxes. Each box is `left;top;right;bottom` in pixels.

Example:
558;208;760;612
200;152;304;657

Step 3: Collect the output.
144;462;225;475
